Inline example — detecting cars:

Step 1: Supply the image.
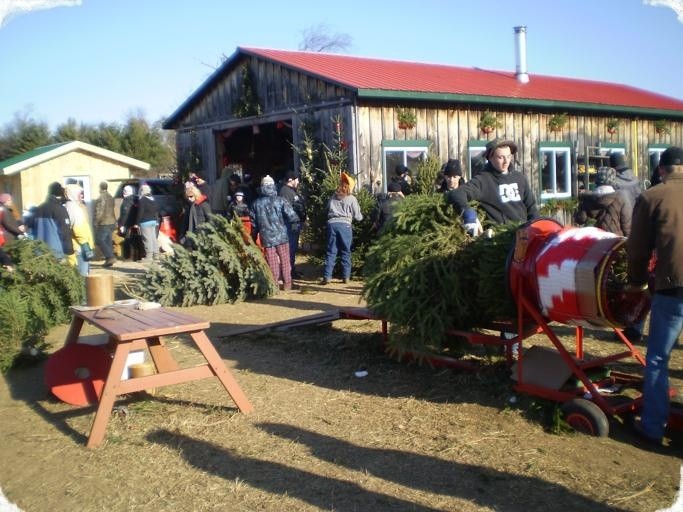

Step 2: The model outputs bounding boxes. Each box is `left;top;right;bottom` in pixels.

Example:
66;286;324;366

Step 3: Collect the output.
115;178;182;220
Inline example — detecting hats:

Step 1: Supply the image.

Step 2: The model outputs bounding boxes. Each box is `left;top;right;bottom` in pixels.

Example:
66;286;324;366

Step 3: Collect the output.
285;171;299;181
261;175;274;185
388;183;401;192
598;167;616;186
0;194;11;203
660;147;683;166
341;172;354;190
48;182;65;196
444;160;462;176
482;139;517;157
396;165;408;176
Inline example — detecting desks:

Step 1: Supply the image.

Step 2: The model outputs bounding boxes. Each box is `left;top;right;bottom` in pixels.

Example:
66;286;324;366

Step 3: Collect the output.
60;299;255;449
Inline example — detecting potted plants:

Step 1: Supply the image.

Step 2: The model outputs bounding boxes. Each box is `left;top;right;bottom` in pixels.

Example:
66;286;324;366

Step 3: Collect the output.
394;105;416;130
653;118;671;135
547;113;567;133
477;107;502;134
605;118;622;134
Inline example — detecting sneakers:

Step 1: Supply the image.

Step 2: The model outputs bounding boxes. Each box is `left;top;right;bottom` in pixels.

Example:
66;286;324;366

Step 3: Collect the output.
105;257;116;265
615;329;643;343
321;280;330;285
343;279;349;284
622;417;662;445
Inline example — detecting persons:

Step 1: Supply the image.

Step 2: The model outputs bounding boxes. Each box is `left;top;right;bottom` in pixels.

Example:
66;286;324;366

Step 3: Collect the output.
573;165;630;236
446;137;541;238
0;167;269;267
278;171;310;281
606;152;644;225
627;146;682;443
247;175;300;292
431;156;472;198
387;163;416;195
318;170;365;285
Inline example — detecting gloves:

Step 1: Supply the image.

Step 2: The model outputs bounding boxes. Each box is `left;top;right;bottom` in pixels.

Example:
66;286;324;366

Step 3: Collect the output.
81;243;96;261
63;254;78;268
463;209;483;237
